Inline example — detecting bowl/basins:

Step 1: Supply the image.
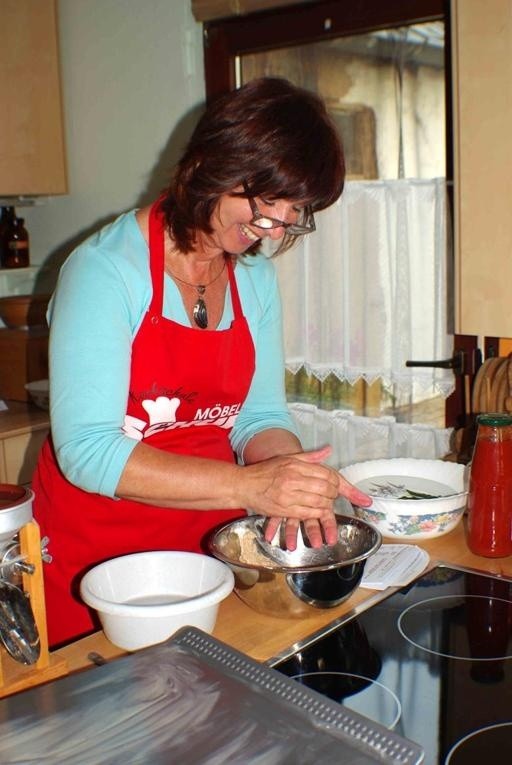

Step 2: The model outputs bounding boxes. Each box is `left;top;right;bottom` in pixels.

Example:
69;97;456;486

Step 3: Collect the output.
23;379;49;410
0;294;52;331
338;457;472;541
79;550;235;654
206;511;384;620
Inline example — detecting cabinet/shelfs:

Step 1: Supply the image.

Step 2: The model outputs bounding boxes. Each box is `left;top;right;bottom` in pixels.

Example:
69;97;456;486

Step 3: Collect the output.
0;1;70;500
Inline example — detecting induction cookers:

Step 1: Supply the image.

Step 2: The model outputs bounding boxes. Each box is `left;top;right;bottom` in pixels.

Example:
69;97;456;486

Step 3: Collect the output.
261;559;512;765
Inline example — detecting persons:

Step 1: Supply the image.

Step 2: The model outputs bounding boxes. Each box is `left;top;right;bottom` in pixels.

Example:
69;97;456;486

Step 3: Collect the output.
28;76;372;652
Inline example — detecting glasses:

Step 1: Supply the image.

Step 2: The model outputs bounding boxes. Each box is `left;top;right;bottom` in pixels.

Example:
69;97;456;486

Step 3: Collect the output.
242;180;316;236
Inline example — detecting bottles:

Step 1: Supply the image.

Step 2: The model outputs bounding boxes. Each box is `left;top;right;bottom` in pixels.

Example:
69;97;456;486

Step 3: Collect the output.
0;206;31;270
465;412;512;558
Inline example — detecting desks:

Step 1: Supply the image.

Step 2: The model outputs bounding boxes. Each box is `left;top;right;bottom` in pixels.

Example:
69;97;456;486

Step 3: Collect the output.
0;500;511;763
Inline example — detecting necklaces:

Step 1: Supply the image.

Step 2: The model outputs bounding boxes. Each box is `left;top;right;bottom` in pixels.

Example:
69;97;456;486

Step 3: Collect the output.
157;252;228;333
